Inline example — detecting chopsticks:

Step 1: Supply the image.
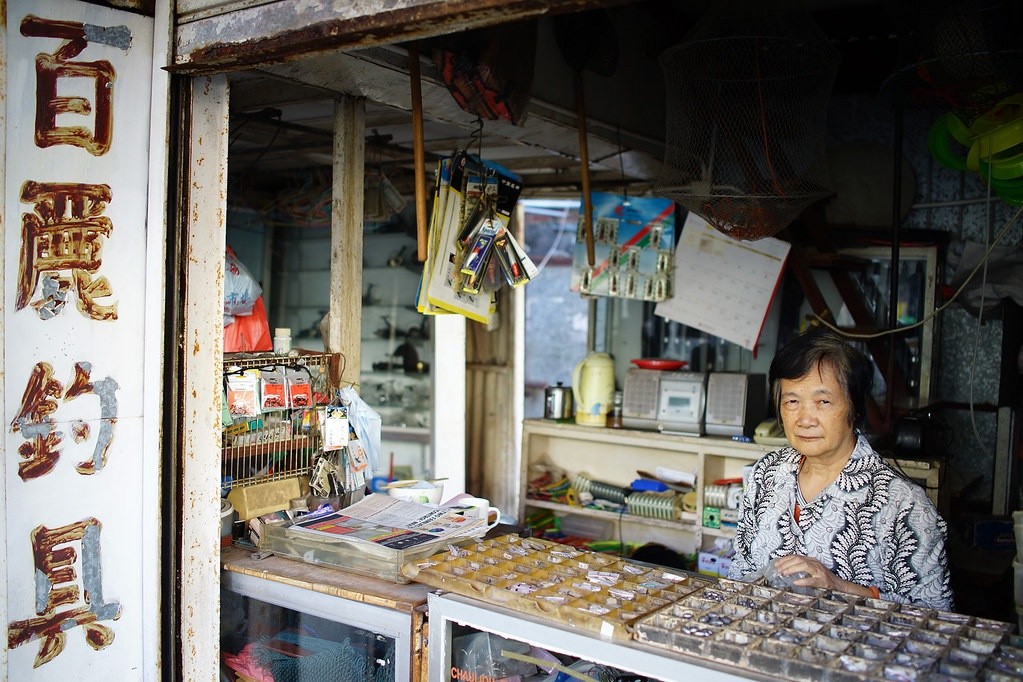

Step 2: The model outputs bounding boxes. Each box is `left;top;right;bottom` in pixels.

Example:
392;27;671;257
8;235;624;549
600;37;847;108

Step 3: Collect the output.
379;478;449;489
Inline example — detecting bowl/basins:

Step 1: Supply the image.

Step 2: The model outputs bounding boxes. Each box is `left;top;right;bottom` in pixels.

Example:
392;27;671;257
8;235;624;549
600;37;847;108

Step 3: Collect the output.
387;479;444;506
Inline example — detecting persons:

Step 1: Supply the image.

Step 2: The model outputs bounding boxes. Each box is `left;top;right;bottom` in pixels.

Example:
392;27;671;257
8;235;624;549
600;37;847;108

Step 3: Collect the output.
724;333;956;614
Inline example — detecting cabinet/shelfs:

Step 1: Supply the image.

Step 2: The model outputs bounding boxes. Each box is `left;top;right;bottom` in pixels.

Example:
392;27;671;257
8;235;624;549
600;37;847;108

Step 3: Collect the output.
519;416;951;580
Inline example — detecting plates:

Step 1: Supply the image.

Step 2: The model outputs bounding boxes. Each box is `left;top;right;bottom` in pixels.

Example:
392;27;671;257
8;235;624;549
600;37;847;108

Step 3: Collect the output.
630;359;688;371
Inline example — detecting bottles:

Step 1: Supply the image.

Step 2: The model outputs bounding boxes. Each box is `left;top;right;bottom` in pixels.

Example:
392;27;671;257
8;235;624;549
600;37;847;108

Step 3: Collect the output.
273;327;292;355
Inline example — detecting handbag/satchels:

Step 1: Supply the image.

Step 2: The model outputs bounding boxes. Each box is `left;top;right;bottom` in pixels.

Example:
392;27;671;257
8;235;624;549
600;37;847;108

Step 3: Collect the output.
224;252;263;316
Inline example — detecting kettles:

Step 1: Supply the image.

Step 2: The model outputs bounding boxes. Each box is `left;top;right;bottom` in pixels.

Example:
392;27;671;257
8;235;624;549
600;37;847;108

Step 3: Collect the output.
574;350;615;414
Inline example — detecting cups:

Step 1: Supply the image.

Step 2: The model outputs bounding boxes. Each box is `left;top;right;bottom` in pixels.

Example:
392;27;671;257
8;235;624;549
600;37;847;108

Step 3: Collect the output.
544;382;573;420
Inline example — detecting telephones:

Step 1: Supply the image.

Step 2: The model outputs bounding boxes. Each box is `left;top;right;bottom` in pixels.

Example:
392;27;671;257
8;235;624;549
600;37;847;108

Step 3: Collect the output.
753;418;789;446
